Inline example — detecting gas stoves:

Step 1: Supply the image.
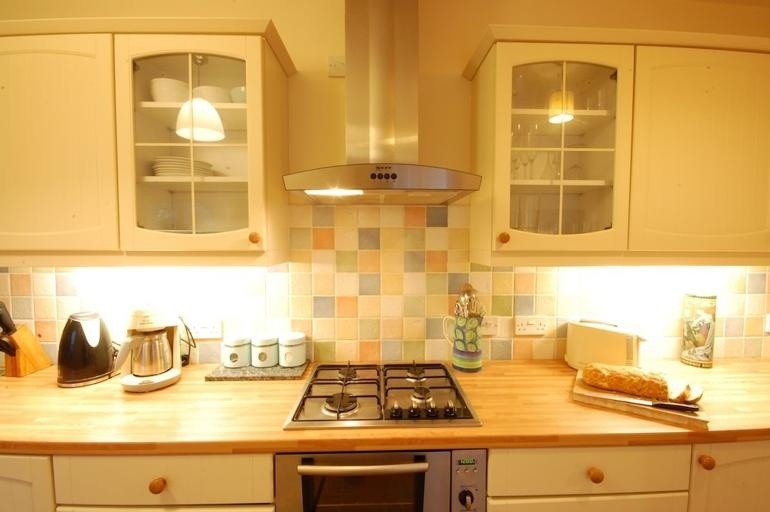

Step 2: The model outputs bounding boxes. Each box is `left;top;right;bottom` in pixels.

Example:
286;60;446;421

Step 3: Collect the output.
283;363;483;426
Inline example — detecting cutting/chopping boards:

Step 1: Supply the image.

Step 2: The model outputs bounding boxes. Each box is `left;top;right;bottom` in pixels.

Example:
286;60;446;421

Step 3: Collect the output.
572;368;711;428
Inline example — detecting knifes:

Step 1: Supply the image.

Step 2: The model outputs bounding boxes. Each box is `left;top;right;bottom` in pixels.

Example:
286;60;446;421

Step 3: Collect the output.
0;300;16;335
584;392;700;412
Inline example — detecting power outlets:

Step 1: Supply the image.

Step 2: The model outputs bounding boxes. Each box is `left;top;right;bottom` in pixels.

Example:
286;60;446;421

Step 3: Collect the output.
515;316;550;336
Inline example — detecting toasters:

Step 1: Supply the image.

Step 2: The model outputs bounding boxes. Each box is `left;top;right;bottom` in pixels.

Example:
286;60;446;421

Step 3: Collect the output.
563;319;647;371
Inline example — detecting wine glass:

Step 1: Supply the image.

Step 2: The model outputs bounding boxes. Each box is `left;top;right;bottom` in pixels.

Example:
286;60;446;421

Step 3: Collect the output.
511;120;541;180
548;145;565;181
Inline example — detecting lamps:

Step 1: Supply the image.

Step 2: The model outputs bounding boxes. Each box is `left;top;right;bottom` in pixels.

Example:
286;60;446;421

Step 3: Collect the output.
176;55;226;143
547;64;575;123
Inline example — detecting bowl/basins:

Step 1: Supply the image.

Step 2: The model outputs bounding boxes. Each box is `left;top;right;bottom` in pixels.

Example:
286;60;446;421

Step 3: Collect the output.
193;85;230;102
148;78;189;102
231;86;247;101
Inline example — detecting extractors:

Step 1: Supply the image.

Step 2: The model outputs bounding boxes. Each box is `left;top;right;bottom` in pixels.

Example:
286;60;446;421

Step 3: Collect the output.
280;0;482;205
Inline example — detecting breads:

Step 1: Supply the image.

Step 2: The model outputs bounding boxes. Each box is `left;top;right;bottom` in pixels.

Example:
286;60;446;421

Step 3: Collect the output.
582;361;703;404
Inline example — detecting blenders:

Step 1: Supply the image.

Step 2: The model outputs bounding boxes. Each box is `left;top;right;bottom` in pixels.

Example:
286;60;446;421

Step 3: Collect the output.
121;308;183;393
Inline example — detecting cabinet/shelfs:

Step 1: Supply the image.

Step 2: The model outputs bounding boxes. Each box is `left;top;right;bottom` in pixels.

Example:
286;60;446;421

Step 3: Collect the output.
52;454;277;512
628;32;769;266
461;23;636;267
110;17;298;266
0;17;120;265
0;452;58;512
688;431;770;512
486;436;691;512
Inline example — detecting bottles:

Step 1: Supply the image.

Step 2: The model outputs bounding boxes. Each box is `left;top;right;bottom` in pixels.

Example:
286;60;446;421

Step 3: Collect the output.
222;332;306;368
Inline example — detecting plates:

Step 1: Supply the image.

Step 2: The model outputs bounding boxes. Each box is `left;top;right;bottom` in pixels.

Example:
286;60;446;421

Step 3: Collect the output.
151;155;214;178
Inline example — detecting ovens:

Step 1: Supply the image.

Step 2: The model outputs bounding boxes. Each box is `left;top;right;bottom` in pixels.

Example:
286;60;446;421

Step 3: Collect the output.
274;448;488;512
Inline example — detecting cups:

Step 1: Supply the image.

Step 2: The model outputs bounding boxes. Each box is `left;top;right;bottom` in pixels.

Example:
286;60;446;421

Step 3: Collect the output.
583;87;607;110
510;195;540;233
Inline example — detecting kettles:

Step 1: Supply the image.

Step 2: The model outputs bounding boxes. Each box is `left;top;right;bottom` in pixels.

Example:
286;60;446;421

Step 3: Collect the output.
56;311;122;389
